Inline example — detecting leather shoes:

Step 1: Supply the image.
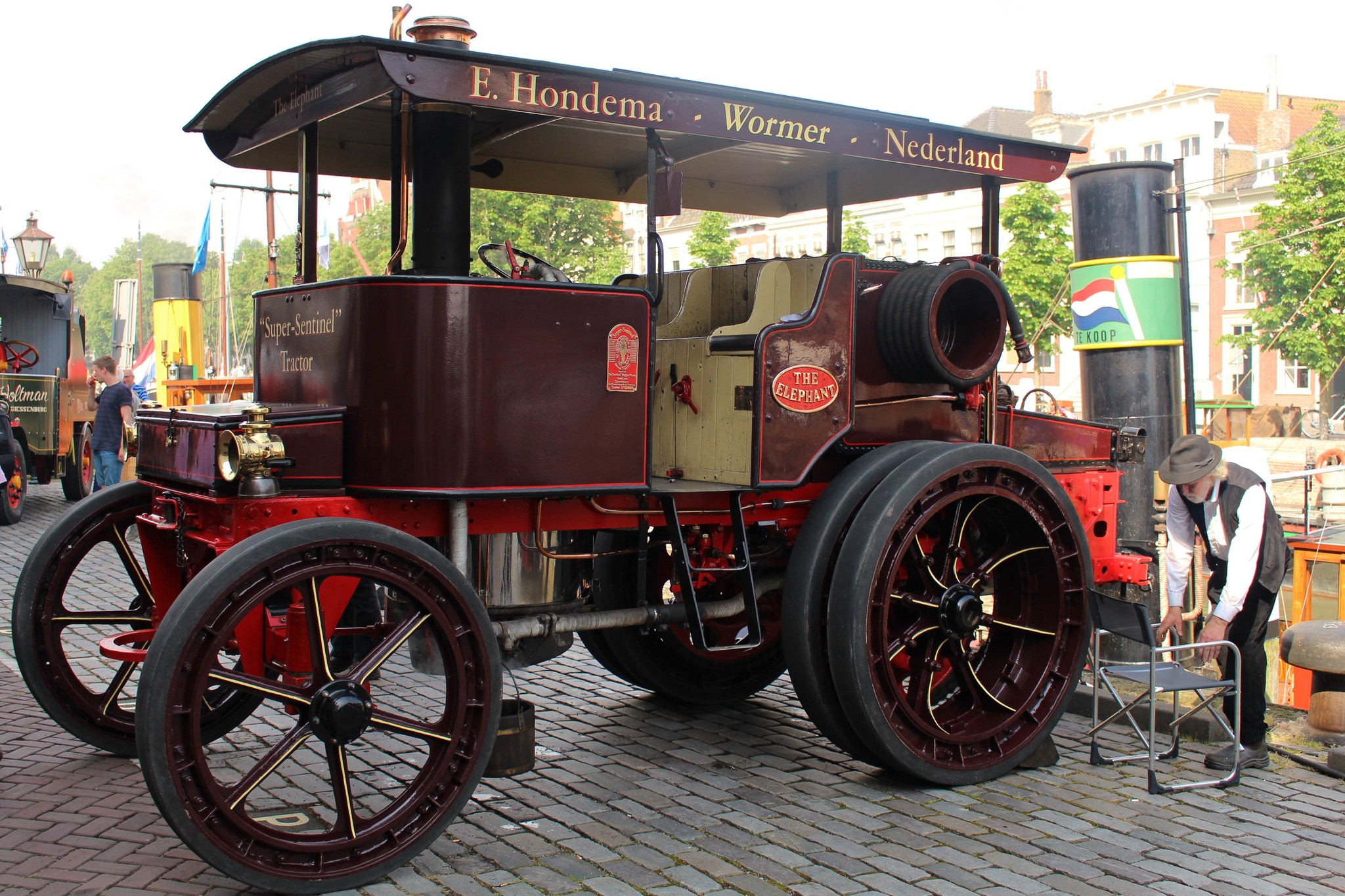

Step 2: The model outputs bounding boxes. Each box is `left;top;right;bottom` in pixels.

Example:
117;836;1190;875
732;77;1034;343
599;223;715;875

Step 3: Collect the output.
1204;733;1269;771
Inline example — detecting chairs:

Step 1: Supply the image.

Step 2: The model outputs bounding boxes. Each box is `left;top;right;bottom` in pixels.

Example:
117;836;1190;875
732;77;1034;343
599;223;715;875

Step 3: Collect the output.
1086;585;1243;795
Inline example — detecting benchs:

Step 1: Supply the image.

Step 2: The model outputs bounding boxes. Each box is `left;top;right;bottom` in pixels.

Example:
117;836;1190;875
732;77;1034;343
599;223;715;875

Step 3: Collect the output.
612;253;859;486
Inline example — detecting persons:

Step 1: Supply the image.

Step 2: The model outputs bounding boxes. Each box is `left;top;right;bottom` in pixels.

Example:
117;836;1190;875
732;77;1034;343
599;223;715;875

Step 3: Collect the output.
1156;434;1292;769
87;356;134;490
115;368;142;425
122;370;150;403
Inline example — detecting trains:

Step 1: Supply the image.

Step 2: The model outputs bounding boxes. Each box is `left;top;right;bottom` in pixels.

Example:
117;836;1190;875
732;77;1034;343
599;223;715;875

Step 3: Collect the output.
0;270;99;527
7;2;1155;896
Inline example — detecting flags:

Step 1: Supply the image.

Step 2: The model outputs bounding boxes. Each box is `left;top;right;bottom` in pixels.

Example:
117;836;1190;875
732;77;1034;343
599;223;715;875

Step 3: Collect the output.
2;227;8;256
317;215;331;270
191;200;210;277
18;263;23;274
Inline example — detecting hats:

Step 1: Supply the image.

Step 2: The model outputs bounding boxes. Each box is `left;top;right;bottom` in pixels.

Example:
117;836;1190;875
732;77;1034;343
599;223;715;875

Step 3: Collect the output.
1159;434;1222;485
